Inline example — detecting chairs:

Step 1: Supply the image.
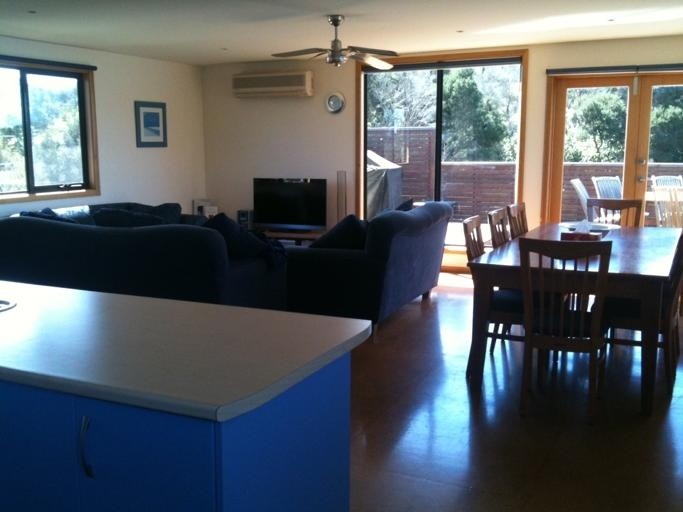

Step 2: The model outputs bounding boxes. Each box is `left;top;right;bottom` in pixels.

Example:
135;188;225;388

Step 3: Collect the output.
507;202;528;239
518;237;612;416
487;206;570;340
569;174;683;228
591;230;683;395
463;214;560;367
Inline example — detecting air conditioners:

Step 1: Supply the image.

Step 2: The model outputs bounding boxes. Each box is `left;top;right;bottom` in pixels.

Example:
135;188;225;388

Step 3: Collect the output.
231;69;314;97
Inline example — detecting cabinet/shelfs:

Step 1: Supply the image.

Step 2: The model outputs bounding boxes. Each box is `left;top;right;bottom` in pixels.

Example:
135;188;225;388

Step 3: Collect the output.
0;353;373;512
262;232;323;246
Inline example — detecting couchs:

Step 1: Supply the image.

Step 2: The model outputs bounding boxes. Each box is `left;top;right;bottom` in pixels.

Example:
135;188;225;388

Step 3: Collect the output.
0;203;287;311
284;198;453;337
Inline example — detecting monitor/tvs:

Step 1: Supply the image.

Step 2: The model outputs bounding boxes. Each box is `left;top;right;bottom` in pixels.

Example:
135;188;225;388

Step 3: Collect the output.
254;178;327;233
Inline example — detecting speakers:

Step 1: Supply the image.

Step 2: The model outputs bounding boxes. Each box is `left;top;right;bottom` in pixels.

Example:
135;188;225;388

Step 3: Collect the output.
337;171;346;223
237;209;253;232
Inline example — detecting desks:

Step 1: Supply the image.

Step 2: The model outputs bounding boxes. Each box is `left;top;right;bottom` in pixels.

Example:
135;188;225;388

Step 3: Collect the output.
467;223;683;417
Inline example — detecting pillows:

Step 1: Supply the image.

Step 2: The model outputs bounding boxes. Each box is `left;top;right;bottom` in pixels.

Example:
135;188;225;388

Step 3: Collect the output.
395;199;414;211
307;215;366;250
20;203;267;258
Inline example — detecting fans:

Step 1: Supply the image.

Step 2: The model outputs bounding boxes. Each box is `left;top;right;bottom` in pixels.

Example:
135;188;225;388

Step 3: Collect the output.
270;15;399;71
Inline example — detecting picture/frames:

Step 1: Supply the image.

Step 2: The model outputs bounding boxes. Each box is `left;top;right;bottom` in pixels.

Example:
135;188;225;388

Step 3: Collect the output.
134;100;168;148
